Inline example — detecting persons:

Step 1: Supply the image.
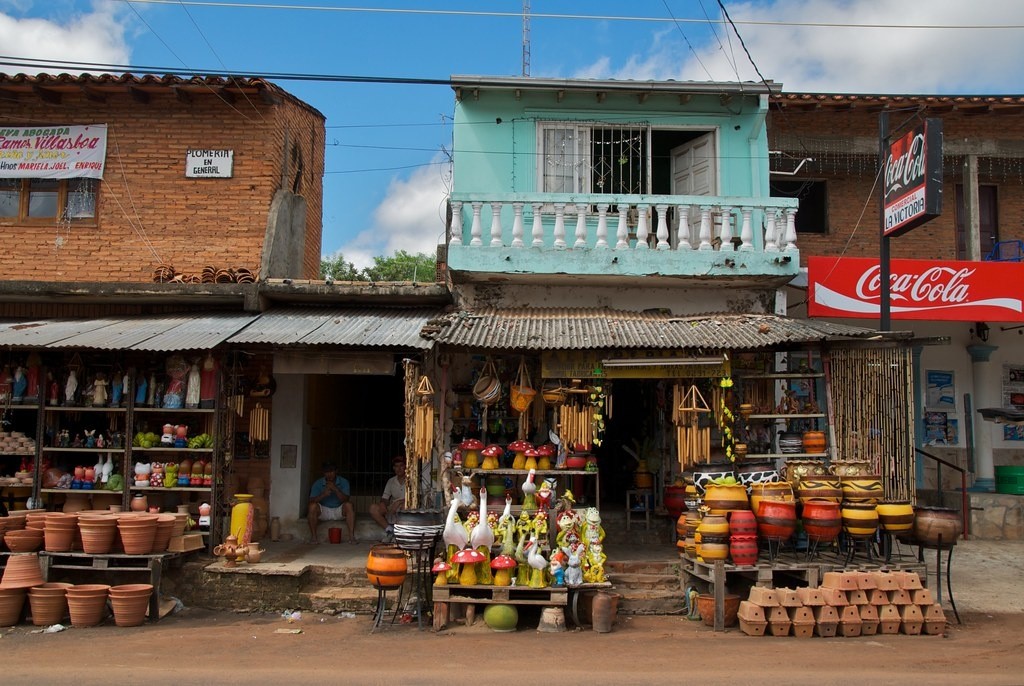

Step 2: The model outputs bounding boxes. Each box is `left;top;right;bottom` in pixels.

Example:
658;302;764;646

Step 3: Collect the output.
307;461;360;545
368;456;406;543
12;366;26;397
48;371;129;406
136;353;217;409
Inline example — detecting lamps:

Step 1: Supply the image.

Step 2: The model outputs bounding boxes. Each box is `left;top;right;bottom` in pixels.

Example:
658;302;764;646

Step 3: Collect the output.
977;321;990;341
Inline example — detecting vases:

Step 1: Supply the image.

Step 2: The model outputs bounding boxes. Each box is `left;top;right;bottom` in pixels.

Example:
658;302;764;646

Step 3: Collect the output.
777;430;803;454
536;606;568;632
695;594;741;628
213;470;281;563
328;528;343;545
0;490;210;627
366;543;407;590
738;571;946;636
473;376;501;404
663;460;962;565
393;508;445;550
803;431;826;453
510;386;536;412
570;587;621;633
541;383;567;406
453;397;472;418
736;442;747;462
486;474;545;505
483;605;518;633
636;461;652;489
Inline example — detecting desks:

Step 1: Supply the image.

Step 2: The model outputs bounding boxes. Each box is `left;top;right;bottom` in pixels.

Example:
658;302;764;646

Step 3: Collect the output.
447;468;599;516
0;549;199;623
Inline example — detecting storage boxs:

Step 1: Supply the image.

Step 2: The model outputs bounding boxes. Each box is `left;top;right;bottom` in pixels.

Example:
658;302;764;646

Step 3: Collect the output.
994;465;1024;495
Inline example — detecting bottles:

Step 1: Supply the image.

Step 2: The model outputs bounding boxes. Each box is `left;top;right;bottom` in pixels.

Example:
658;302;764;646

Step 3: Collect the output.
636;459;652;496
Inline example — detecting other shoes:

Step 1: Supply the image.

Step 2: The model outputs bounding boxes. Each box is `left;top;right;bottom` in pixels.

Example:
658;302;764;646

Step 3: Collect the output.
382;532;393;543
391;537;397;543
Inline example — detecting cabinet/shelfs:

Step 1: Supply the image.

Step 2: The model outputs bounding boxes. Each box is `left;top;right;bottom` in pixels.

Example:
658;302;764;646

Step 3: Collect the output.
626;491;656;531
450;416;526;468
0;364;235;558
432;506;568;632
732;371;837;461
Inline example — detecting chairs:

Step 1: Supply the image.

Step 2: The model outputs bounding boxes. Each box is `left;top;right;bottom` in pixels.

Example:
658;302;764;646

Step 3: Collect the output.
985;239;1024;262
710;211;742;251
627;210;656;249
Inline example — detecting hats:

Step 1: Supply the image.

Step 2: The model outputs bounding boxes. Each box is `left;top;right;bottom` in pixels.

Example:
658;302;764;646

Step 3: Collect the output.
392;456;405;463
322;462;339;473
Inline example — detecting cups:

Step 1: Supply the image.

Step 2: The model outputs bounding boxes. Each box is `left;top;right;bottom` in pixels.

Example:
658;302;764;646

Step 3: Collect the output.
329;527;342;544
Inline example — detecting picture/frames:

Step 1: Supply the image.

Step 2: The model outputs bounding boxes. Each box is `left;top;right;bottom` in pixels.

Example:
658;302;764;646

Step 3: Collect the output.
280;445;297;468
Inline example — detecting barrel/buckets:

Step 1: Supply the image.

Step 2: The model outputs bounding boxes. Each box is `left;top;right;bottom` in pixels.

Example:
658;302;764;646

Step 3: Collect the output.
585;592;620;626
511;386;536;411
